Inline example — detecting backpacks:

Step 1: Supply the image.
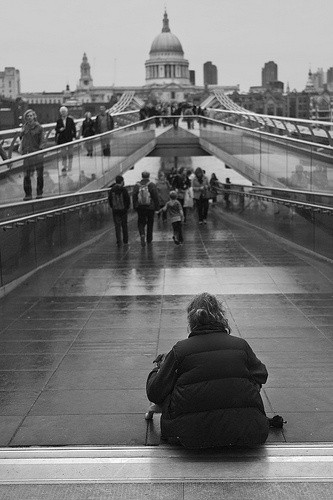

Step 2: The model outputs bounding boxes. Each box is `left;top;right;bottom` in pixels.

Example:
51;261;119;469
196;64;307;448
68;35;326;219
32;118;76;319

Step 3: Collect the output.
136;181;152;205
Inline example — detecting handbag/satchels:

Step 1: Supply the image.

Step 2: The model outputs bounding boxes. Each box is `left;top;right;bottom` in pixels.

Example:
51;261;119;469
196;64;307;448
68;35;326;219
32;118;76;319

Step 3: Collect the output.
17;138;24;155
202;185;216;199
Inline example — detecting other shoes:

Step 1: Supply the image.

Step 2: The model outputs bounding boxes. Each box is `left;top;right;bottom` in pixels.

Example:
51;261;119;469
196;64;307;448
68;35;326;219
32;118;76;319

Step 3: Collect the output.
35;194;42;198
23;196;32;201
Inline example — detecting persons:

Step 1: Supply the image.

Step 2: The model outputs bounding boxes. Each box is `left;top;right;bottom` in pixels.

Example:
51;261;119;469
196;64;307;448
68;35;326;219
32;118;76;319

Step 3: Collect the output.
108;176;130;246
82;113;95;157
156;171;171;223
133;172;161;246
224;177;231;207
167;168;195;221
20;109;45;201
96;106;114;156
146;292;269;451
192;168;210;225
55;106;77;172
156;190;185;245
140;105;208;129
209;173;219;202
